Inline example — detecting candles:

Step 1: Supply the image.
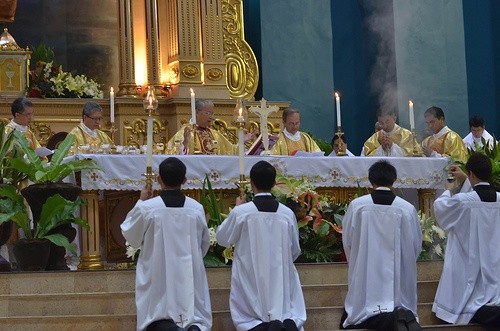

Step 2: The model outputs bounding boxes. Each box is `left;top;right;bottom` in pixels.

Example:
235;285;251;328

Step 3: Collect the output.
146;98;153;167
110;86;114;123
408;100;415;130
334;92;342;127
237;108;245;173
190;88;196;125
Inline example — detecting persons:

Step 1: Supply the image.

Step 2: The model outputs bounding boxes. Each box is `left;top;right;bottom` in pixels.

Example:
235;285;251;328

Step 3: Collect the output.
66;101;116;152
215;161;307;331
4;97;49;197
328;135;354;157
120;157;213;331
361;105;423;157
166;99;256;154
417;106;467;161
341;160;423;331
432;152;500;331
462;115;499;152
270;109;321;155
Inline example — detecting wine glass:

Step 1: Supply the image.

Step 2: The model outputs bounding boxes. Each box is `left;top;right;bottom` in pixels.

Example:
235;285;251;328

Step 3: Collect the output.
76;143;164;155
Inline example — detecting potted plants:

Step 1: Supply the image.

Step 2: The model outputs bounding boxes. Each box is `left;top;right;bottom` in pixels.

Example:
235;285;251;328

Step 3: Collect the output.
0;119;103;272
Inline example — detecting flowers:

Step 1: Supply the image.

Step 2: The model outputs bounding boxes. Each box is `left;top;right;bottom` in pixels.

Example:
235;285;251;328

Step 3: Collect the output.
126;170;447;260
26;45;105;101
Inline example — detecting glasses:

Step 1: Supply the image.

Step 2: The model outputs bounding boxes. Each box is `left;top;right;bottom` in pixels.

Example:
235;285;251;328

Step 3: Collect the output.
84;113;103;120
200;110;215;117
16;111;35;116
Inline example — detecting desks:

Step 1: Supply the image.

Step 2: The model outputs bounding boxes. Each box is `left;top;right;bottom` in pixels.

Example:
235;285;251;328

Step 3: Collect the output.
32;151;451;270
